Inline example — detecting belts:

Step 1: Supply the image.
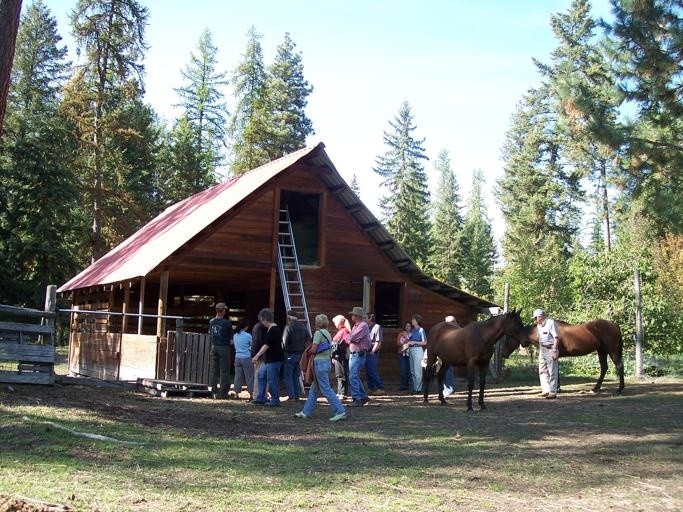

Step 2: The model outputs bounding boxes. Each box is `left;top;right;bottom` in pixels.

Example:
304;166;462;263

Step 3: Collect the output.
350;352;356;354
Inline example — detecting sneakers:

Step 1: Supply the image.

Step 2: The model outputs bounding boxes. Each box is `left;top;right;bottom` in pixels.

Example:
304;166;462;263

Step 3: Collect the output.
546;395;556;399
264;403;280;407
294;411;312;418
538;393;549;397
347;400;363;407
251;401;265;405
329;414;347;421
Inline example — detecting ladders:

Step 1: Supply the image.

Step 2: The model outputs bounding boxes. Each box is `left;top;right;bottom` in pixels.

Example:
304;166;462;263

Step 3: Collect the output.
276;205;317;395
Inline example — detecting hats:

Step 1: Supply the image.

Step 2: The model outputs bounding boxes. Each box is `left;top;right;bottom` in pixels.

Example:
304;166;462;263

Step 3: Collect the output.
349;307;366;318
531;309;544;319
216;303;228;310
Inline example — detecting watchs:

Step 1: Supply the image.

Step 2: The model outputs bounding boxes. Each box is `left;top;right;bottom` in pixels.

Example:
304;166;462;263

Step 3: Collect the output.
551;349;558;352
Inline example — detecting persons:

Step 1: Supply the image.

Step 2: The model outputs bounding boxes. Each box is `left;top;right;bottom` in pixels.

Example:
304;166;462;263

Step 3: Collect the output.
533;309;559;399
366;312;387;396
251;309;283;407
332;315;351;398
345;306;370;407
209;303;234;399
400;314;428;394
282;311;311;401
251;323;272;400
233;319;255;402
396;321;412;390
294;314;347;422
440;316;460;397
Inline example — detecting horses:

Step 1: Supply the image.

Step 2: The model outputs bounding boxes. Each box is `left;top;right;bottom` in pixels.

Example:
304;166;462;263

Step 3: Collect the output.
417;307;529;414
501;319;625;396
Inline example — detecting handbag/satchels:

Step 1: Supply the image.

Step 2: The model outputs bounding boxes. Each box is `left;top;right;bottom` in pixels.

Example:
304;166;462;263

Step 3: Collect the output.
316;329;331;353
